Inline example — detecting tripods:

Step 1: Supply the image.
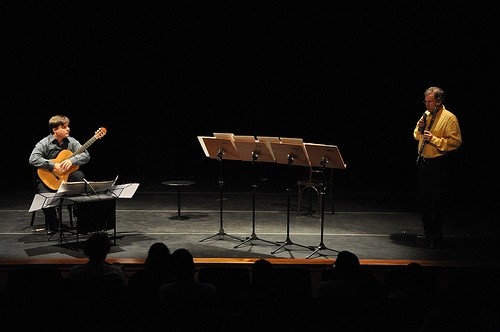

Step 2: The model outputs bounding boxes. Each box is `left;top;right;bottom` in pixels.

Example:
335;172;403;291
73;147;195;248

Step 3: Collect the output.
199;137;347;260
41;194;83;241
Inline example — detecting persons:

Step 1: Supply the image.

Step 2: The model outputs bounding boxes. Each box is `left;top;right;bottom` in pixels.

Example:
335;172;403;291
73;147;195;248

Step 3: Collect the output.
29;115;90;234
412;86;462;248
65;233;500;332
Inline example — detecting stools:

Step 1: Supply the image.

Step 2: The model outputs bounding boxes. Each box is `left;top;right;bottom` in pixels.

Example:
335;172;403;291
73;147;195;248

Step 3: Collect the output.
163;180;196;220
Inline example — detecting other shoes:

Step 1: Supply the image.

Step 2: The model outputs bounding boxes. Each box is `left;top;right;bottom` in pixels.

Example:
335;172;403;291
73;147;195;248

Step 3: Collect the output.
416;235;427;241
427;244;433;248
47;230;55;235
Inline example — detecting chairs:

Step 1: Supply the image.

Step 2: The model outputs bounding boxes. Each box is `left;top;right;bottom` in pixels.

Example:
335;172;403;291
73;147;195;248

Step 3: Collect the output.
30;166;73;231
297;166;334;219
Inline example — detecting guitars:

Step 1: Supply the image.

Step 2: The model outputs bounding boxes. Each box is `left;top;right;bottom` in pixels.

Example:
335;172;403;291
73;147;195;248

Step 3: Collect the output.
35;127;107;192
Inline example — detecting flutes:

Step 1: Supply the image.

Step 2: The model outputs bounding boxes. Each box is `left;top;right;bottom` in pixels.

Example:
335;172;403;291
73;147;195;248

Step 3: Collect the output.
415;103;439;165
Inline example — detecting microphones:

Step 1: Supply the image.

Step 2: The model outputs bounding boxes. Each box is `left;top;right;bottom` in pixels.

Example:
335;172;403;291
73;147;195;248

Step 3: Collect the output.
422;113;427;135
